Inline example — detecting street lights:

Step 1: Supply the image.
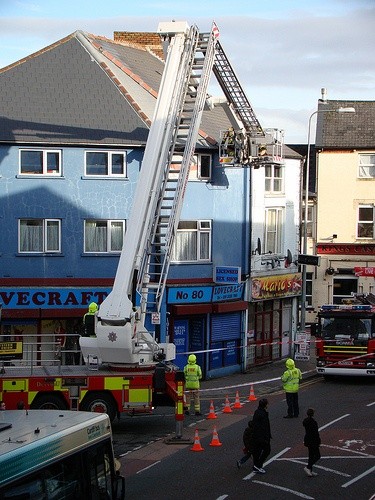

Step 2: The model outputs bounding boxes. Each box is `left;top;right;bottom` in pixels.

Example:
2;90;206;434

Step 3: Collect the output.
301;107;357;331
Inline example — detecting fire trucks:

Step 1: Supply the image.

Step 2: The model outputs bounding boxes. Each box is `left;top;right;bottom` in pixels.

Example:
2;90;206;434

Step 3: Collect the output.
0;20;284;431
310;292;375;383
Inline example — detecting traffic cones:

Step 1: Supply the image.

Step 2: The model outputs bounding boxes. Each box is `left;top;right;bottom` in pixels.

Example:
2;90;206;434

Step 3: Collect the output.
206;399;218;419
233;391;242;409
209;425;222;446
248;384;257;401
190;428;205;451
223;395;233;413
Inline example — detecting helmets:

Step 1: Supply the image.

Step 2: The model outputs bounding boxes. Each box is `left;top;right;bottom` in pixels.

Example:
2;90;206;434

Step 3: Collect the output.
285;359;295;369
188;354;196;364
88;302;98;313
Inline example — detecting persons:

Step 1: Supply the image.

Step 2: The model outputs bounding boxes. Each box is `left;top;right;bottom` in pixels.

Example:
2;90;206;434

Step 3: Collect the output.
184;354;202;415
281;359;302;418
82;302;98;336
302;408;321;477
220;128;268;165
237;398;272;473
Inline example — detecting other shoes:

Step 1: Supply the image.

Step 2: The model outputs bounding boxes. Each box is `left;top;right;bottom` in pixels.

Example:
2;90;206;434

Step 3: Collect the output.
304;466;312;476
293;414;298;418
254;465;265;473
253;469;259;472
237;461;241;471
283;416;289;418
185;411;189;415
311;470;318;476
195;412;202;416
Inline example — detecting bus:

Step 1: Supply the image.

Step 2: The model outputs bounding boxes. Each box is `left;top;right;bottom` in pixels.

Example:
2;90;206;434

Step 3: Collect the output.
0;408;126;500
